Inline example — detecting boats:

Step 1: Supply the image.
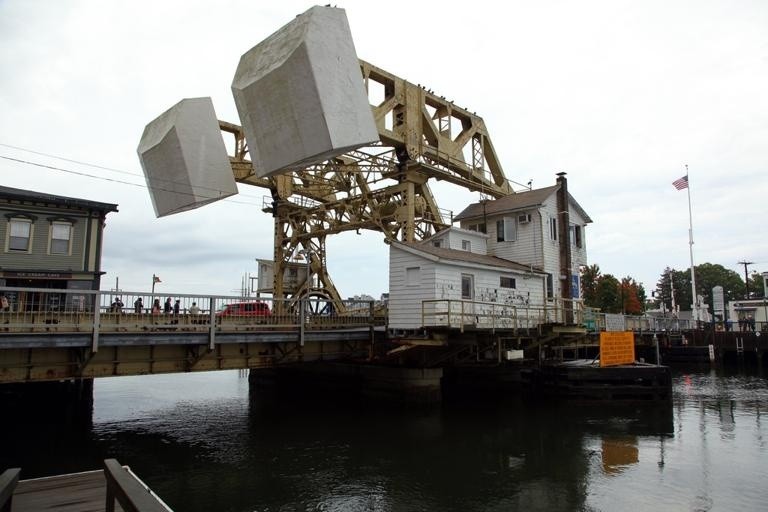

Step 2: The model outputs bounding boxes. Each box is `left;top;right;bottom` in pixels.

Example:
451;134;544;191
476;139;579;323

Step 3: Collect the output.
552;357;672;398
0;457;172;512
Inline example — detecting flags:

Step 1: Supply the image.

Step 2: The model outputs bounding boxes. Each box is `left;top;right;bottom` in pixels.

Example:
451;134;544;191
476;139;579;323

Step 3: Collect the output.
672;174;690;191
153;277;161;282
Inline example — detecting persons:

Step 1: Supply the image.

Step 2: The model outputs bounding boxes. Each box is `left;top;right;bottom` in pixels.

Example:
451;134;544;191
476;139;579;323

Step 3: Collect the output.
164;297;173;313
134;297;143;313
0;294;10;324
152;299;162;313
173;300;180;313
110;297;124;312
189;302;201;314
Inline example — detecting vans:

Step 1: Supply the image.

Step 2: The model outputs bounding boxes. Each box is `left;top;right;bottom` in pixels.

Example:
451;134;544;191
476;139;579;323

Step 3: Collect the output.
216;301;270;316
